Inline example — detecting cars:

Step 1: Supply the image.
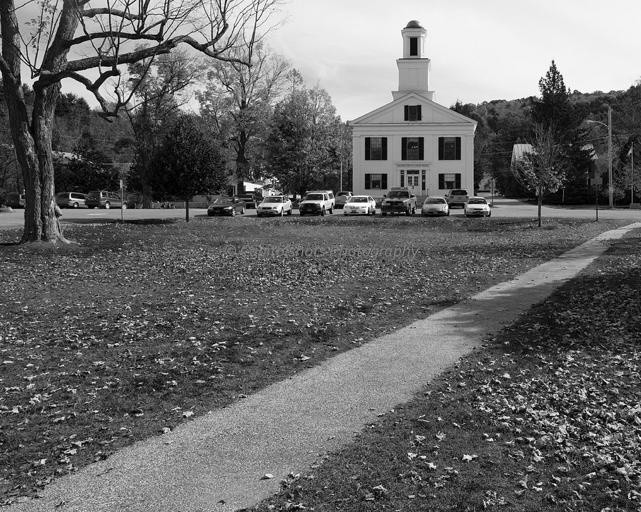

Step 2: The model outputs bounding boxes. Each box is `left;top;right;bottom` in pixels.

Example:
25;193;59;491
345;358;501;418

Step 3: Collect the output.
381;188;491;216
299;191;376;217
239;194;257;209
208;196;247;217
257;196;292;217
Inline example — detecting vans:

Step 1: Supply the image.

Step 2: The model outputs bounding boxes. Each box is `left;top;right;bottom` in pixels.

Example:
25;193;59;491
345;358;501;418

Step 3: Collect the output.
85;190;128;209
56;192;88;209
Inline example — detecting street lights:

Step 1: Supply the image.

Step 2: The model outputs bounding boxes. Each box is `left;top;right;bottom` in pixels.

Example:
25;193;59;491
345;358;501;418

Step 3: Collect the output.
587;120;614;208
